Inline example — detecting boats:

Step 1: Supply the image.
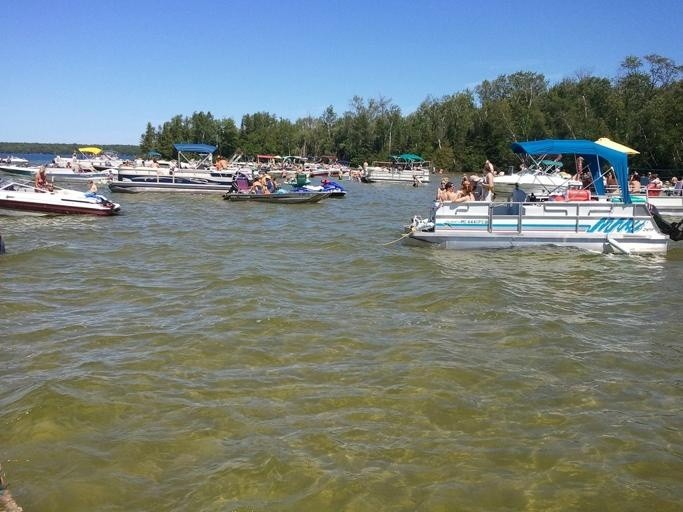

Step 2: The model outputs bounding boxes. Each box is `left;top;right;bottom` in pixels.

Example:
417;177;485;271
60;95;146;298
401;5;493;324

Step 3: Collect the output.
222;163;333;205
0;143;348;193
358;152;433;183
0;174;122;217
403;138;683;253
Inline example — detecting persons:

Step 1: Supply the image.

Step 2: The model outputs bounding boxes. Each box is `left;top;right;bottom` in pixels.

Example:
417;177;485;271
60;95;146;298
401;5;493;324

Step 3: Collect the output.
134;156;160;168
436;160;494;203
215;155;228;170
576;156;679;196
35;167;53;193
229;176;239;192
85;180;97;197
251;170;277;195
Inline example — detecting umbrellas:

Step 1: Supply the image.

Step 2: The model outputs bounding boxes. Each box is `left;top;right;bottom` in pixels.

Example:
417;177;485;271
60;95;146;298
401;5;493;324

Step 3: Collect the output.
594;138;640;155
